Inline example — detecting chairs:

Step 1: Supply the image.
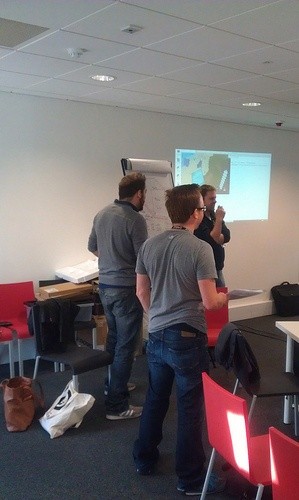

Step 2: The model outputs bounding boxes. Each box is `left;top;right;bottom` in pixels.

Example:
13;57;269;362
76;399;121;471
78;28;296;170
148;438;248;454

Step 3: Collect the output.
232;323;299;437
0;326;15;380
205;287;230;369
31;302;113;395
0;281;37;377
200;372;273;500
268;427;299;500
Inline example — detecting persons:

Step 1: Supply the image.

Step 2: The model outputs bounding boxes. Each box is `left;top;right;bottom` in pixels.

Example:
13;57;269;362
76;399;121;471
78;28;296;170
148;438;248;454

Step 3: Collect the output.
193;184;230;288
87;172;148;419
131;185;228;495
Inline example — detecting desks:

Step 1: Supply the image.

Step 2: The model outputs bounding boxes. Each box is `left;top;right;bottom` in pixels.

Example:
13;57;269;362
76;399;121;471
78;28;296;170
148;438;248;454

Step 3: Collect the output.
276;321;299;425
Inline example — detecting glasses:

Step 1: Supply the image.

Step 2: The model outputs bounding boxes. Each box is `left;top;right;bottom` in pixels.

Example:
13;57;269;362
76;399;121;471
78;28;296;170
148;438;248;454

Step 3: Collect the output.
197;205;207;212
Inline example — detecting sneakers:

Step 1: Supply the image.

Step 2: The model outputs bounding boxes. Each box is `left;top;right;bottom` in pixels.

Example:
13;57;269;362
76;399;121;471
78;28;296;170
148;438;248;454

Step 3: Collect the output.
106;406;144;419
104;383;136;394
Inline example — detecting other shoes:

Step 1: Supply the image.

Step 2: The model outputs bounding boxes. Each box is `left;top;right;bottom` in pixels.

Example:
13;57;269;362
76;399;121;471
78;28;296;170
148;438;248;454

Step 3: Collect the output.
176;475;224;495
133;461;153;475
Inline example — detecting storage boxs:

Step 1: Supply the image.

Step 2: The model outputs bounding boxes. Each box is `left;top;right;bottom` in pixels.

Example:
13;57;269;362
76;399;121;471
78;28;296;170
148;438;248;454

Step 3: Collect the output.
37;283;92;305
54;260;100;283
79;315;108;346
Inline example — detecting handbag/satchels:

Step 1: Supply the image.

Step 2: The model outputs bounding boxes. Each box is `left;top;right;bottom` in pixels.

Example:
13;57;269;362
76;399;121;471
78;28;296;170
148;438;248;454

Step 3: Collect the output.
271;281;299;317
0;377;44;432
38;380;96;438
